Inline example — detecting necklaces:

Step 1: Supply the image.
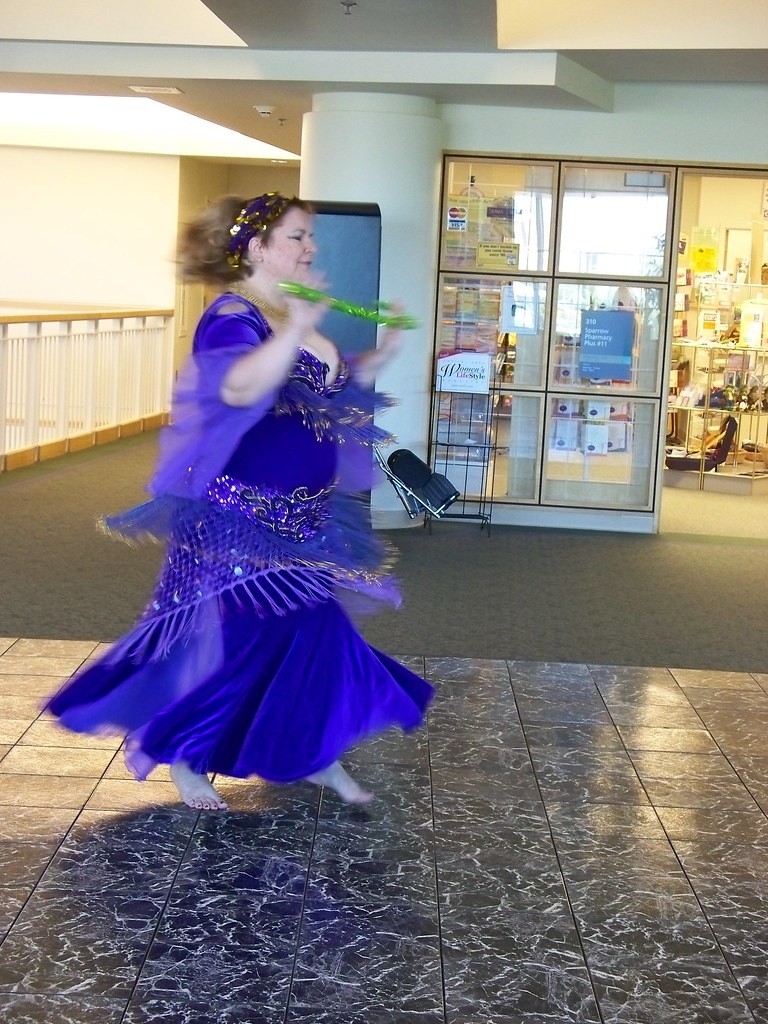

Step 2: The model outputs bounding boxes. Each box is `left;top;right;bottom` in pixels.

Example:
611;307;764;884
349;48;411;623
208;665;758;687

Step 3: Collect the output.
227;277;290;324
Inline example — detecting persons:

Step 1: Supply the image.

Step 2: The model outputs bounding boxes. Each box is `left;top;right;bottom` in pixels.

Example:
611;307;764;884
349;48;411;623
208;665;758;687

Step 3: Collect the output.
45;190;437;810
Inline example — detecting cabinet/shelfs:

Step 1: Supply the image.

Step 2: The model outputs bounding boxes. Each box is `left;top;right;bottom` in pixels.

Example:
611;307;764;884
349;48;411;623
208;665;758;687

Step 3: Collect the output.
663;342;768;496
